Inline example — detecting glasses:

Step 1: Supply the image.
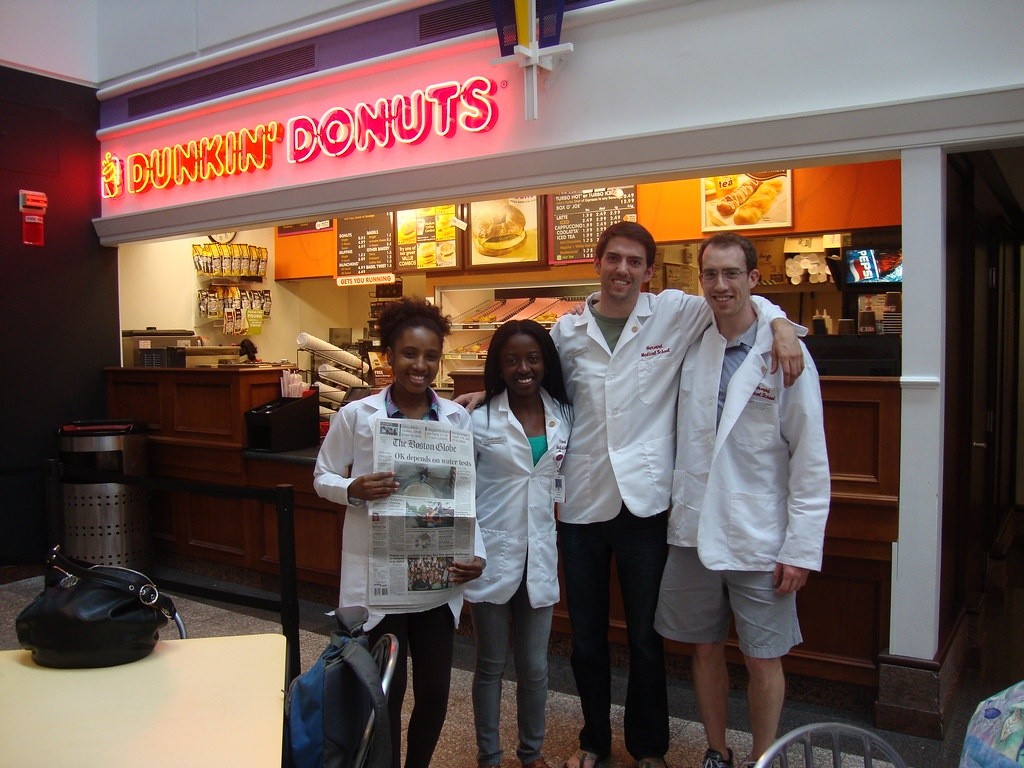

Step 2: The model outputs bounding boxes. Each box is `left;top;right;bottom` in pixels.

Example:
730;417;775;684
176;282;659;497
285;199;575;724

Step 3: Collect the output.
699;269;750;282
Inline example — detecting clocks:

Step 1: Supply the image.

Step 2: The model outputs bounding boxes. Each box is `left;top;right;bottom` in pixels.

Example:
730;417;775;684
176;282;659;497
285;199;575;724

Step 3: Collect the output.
208;232;237;245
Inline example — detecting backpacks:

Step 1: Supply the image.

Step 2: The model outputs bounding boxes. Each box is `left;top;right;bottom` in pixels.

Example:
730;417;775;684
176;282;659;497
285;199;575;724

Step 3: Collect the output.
282;606;393;768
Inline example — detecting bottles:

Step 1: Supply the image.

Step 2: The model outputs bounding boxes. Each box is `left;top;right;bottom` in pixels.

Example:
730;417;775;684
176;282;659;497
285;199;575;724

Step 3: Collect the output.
811;309;833;334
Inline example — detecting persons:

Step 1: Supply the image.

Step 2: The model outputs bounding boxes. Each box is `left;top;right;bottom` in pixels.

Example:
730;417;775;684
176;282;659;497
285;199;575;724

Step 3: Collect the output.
461;318;576;768
314;301;487;768
453;222;809;768
408;557;454;590
566;233;831;768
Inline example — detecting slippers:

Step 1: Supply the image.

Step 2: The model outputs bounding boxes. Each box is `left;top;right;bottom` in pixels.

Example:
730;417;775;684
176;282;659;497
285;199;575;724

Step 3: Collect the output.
637;757;668;768
562;750;599;768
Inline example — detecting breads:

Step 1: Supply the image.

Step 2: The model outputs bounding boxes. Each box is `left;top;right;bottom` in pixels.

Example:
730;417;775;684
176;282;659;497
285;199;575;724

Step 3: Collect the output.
473;204;527;257
716;177;782;225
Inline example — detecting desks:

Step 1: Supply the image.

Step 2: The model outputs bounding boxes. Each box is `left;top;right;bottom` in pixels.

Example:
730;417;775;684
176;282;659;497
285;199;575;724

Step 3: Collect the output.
0;633;292;768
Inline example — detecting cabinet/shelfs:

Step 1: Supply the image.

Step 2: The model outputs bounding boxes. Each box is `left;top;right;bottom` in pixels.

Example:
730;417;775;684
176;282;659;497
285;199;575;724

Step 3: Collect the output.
430;278;605;390
194;276;272;328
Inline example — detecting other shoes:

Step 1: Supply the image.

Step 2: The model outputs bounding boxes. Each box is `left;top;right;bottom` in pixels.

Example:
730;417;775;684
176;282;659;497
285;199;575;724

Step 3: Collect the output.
702;748;733;768
524;757;551;768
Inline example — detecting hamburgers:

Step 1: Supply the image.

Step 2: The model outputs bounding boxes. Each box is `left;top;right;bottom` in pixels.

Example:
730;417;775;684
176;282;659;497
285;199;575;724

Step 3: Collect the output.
440;242;455;261
419;244;434;263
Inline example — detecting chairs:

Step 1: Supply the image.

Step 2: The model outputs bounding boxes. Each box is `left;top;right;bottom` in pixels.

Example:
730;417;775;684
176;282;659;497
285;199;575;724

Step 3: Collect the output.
351;633;399;768
753;723;910;768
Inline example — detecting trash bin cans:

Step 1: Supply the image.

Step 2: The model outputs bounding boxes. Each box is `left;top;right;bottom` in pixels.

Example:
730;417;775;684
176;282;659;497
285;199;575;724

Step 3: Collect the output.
57;418;154;581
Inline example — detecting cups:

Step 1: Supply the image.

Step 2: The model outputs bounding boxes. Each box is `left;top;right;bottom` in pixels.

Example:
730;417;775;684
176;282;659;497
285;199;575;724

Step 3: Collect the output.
317;362;368;389
857;310;876;337
837;319;854;335
881;291;902;334
296;332;370;374
313;381;346;420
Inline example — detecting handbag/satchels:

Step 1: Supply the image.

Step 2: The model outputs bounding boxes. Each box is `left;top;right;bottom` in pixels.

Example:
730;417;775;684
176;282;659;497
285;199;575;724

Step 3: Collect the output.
15;545;177;669
